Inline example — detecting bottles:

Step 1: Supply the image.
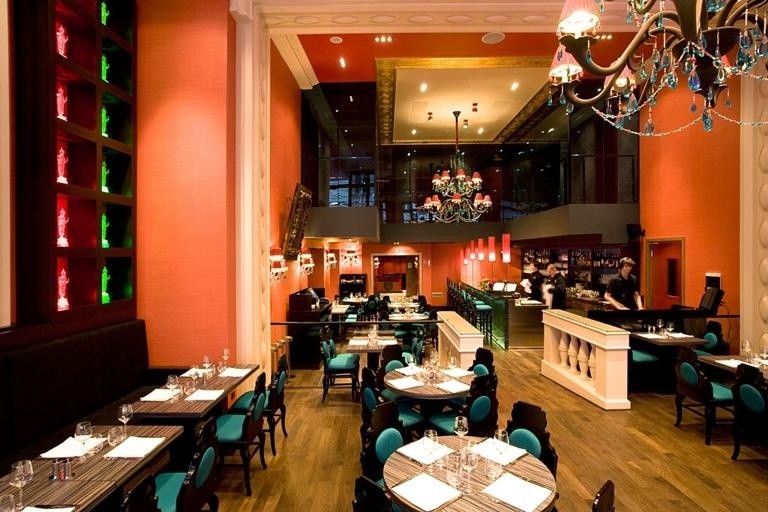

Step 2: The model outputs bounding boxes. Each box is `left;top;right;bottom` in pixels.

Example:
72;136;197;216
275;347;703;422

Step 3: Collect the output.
524;250;550;263
571;249;591;266
594;248;620;268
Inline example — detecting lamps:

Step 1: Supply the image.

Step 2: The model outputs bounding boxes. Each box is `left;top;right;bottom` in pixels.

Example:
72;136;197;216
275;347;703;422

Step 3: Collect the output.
422;110;493;225
460;232;511;294
541;2;768;137
343;249;358;267
297;247;315;276
325;248;337;272
269;246;290;283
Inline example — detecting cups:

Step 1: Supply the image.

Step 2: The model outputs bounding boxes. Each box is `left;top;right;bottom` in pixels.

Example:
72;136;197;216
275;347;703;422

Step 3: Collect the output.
484;455;502;480
107;428;124;446
647;324;655;335
183;381;195;395
446;452;463;485
85;434;104;453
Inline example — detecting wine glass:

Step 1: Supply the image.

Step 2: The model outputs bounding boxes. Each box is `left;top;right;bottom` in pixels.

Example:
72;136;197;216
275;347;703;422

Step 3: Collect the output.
657;319;663;335
200;352;213;379
741;338;751;364
0;495;15;512
166;375;178;399
493;430;509;470
422;430;438;473
459;447;478;497
665;322;675;341
447;356;457;377
74;421;92;463
116;404;133;440
10;459;33;510
406;355;415;377
453;415;468;453
221;347;231;369
421;359;433;388
189;365;199;386
758;345;767;370
431;350;439;381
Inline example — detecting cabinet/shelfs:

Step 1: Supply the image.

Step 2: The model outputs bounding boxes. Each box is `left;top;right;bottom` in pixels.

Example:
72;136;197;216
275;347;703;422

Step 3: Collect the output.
520;243;620;298
5;0;142;335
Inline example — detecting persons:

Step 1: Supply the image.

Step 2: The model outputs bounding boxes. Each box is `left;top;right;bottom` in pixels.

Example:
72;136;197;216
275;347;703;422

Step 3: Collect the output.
523;261;544;301
543;263;567;310
603;257;643;310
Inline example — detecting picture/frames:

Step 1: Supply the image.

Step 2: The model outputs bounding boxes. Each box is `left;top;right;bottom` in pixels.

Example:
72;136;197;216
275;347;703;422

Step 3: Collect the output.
283;181;314;261
